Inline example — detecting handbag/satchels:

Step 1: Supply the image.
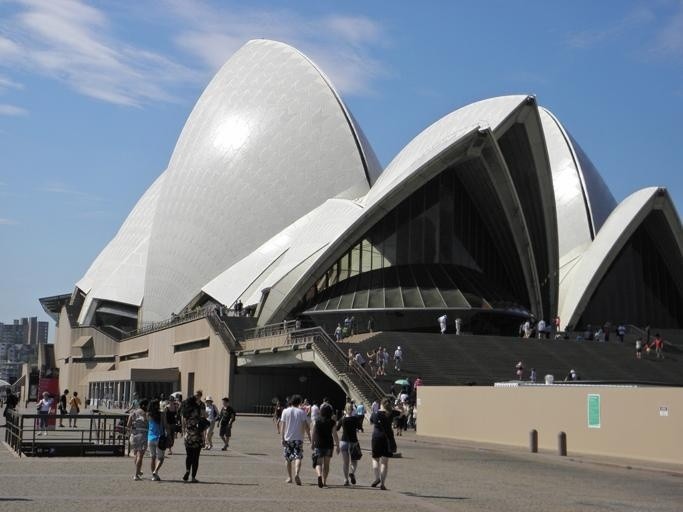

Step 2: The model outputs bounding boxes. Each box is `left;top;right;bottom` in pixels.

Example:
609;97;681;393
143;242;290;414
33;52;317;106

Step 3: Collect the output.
158;434;167;450
386;435;396;454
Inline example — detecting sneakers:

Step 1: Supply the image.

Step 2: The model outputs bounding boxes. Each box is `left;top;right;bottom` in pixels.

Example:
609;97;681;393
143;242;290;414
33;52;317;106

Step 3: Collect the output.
349;473;356;484
318;476;323;488
192;480;199;482
372;479;380;487
295;475;301;485
286;477;292;483
134;471;161;481
344;484;349;485
381;487;386;490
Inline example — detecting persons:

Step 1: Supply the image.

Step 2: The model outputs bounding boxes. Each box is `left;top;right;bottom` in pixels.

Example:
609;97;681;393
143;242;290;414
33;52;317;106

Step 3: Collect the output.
543;370;555;384
515;359;528;381
333;315;376;341
528;367;538;383
510;315;672;361
274;377;424;490
348;345;403;379
171;300;243;321
34;390;56;437
570;369;578;380
454;317;465;336
116;391;236;483
436;311;449;337
2;386;20;420
58;387;70;428
67;390;83;428
283;316;303;332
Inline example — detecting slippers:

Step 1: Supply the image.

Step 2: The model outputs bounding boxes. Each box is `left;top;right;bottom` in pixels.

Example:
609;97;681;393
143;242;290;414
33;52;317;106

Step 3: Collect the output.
183;472;190;481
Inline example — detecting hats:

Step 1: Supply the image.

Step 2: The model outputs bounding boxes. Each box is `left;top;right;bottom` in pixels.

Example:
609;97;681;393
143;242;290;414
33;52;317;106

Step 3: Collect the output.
205;396;214;403
